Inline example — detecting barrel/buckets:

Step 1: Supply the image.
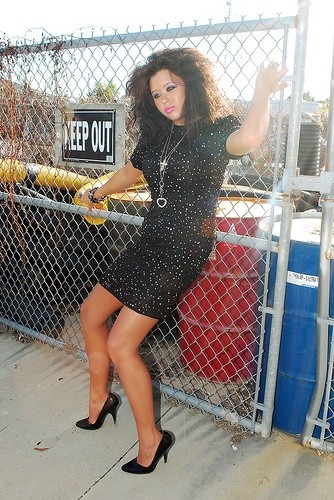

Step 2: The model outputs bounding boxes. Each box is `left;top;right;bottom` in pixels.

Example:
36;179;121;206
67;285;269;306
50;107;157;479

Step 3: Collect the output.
284;123;321;176
107;188;334;437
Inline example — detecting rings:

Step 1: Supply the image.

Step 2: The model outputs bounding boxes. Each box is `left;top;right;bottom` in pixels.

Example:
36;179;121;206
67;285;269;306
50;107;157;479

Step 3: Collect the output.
77;192;82;198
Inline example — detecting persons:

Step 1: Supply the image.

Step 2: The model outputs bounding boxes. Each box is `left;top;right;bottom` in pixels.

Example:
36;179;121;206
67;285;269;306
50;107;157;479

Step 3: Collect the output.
73;47;288;475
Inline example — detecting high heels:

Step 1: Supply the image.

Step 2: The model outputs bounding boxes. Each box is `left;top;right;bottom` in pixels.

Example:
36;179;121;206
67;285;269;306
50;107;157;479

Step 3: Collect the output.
122;429;174;474
76;393;119;431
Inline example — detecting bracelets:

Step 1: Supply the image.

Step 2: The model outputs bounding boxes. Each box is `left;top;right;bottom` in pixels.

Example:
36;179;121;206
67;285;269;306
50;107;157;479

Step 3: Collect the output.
87;187;105;203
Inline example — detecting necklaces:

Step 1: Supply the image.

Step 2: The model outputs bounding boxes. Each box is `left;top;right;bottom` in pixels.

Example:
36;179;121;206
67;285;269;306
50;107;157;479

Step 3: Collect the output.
156;119;194;208
